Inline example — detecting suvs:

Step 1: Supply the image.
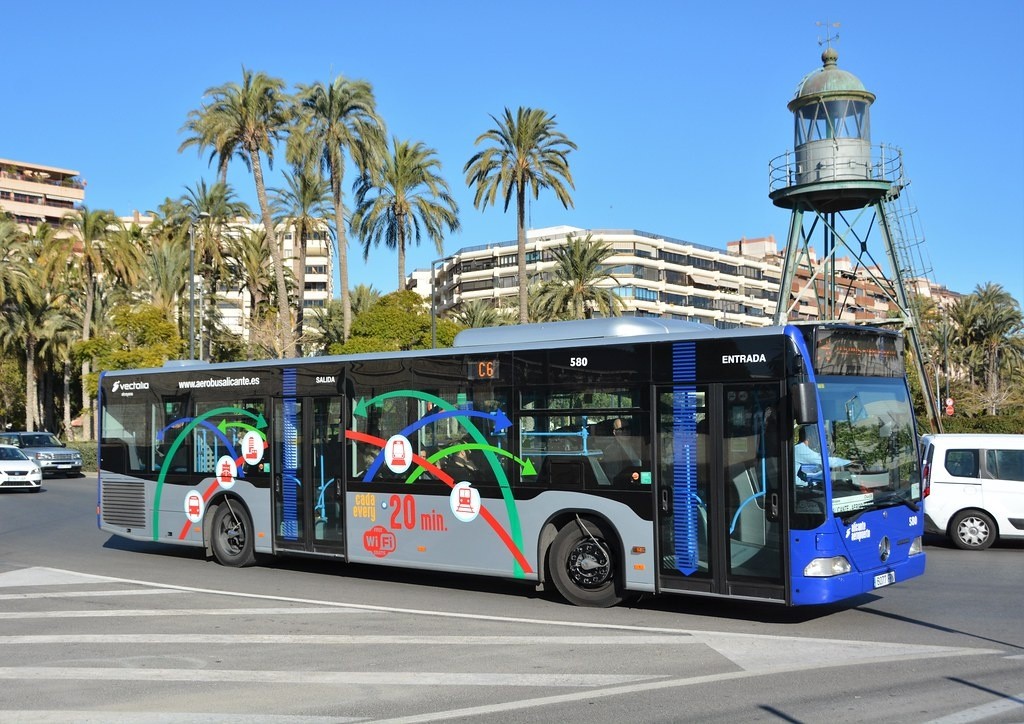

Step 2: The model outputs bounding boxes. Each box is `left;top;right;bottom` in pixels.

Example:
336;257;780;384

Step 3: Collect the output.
0;431;83;479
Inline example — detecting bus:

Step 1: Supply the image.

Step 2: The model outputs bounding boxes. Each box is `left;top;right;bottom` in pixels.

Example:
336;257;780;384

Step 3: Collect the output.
96;317;929;609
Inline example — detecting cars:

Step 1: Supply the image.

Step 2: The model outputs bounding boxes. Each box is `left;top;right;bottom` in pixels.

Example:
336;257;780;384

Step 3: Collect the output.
0;444;43;494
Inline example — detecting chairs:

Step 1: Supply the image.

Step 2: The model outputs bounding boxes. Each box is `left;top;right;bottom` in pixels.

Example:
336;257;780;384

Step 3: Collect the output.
759;410;780;490
101;418;649;484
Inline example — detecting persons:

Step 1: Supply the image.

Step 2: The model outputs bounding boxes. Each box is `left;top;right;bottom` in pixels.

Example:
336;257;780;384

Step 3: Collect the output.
794;423;860;504
448;439;490;481
362;440;394;480
602;416;641;485
412;444;442;482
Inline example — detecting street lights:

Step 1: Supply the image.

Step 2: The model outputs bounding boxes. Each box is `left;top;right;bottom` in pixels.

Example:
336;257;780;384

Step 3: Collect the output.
431;253;461;349
188;212;211;359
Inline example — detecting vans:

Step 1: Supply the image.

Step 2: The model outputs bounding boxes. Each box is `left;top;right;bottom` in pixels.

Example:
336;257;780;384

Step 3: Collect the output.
914;433;1024;551
